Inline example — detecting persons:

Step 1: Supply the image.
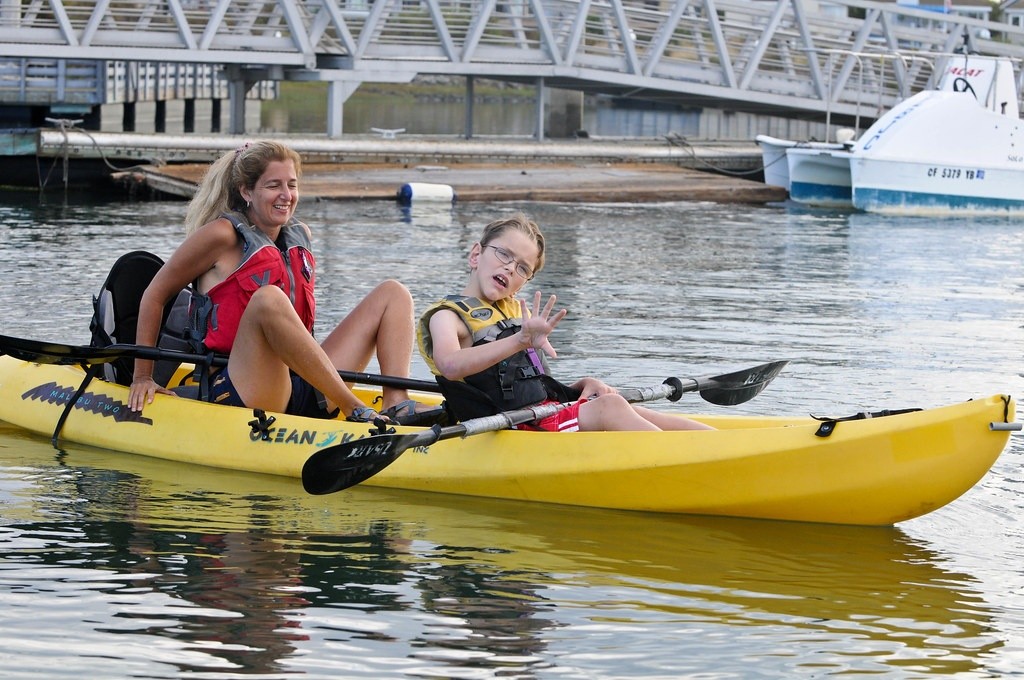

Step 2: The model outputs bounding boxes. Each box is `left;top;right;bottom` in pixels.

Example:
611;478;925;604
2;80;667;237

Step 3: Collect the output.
127;140;436;425
416;210;720;432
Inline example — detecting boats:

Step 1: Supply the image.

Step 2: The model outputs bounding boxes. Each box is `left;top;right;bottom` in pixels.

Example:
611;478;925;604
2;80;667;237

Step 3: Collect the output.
754;46;1023;217
1;353;1023;529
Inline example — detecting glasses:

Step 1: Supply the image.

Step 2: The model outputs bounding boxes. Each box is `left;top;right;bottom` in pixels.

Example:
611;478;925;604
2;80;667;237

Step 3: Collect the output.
482;245;535;281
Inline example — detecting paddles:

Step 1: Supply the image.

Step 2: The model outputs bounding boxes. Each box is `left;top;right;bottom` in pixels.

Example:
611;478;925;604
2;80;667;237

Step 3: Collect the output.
301;360;790;497
0;333;439;393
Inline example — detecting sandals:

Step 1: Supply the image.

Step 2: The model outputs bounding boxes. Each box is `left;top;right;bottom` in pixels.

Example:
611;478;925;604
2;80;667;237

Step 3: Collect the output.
379;400;449;427
346;407;401;425
